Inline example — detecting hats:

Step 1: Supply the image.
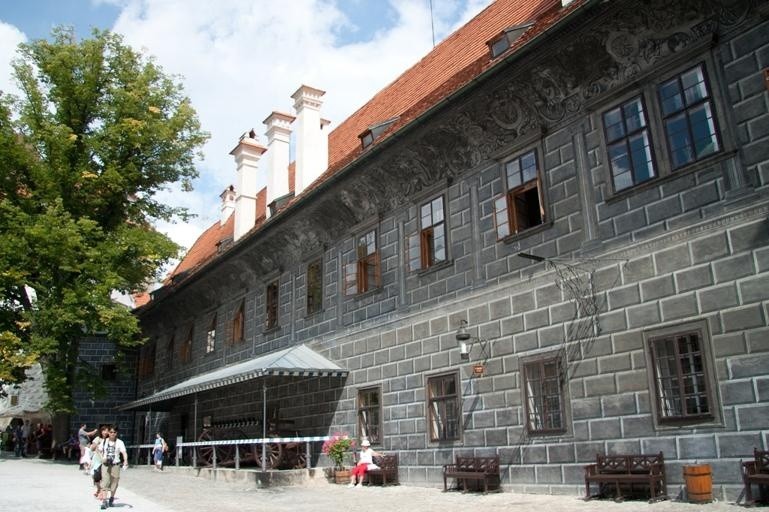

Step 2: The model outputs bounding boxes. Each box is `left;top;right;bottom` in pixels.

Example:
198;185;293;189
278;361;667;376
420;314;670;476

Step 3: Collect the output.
361;440;370;446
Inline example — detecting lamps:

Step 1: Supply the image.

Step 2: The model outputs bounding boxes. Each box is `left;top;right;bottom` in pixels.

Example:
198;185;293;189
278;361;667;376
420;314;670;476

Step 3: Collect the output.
456;319;470;340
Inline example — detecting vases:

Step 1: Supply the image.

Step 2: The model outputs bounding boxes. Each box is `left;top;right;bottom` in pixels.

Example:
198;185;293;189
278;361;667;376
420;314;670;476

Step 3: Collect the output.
334;469;350;484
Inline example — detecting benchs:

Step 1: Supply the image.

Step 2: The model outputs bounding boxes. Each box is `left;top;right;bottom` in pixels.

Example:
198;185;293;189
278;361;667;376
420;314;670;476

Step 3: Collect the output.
354;452;399;487
741;446;769;508
442;454;501;495
583;450;671;504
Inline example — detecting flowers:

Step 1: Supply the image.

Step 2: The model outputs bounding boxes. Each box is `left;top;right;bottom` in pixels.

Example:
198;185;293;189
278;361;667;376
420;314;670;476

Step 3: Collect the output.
322;430;356;470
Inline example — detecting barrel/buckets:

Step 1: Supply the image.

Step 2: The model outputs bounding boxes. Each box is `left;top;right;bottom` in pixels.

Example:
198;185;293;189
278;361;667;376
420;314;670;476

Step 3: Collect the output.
682;463;713;503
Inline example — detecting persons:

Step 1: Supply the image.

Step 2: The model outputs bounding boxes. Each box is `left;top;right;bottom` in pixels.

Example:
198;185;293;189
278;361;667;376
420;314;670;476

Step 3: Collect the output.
346;440;387;488
89;424;109;502
150;432;166;472
5;419;80;460
79;440;92;475
77;422;98;470
96;425;130;509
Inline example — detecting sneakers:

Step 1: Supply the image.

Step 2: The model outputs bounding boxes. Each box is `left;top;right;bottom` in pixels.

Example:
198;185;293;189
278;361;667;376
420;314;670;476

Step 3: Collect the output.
347;483;363;488
100;497;115;509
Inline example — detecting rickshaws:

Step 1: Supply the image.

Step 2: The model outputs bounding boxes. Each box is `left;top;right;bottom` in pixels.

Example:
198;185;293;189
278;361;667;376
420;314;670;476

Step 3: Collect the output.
220;433;303;470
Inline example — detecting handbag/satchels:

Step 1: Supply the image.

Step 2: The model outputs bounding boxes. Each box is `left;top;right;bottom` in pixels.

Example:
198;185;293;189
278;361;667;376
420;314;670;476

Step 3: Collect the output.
93;464;102;482
